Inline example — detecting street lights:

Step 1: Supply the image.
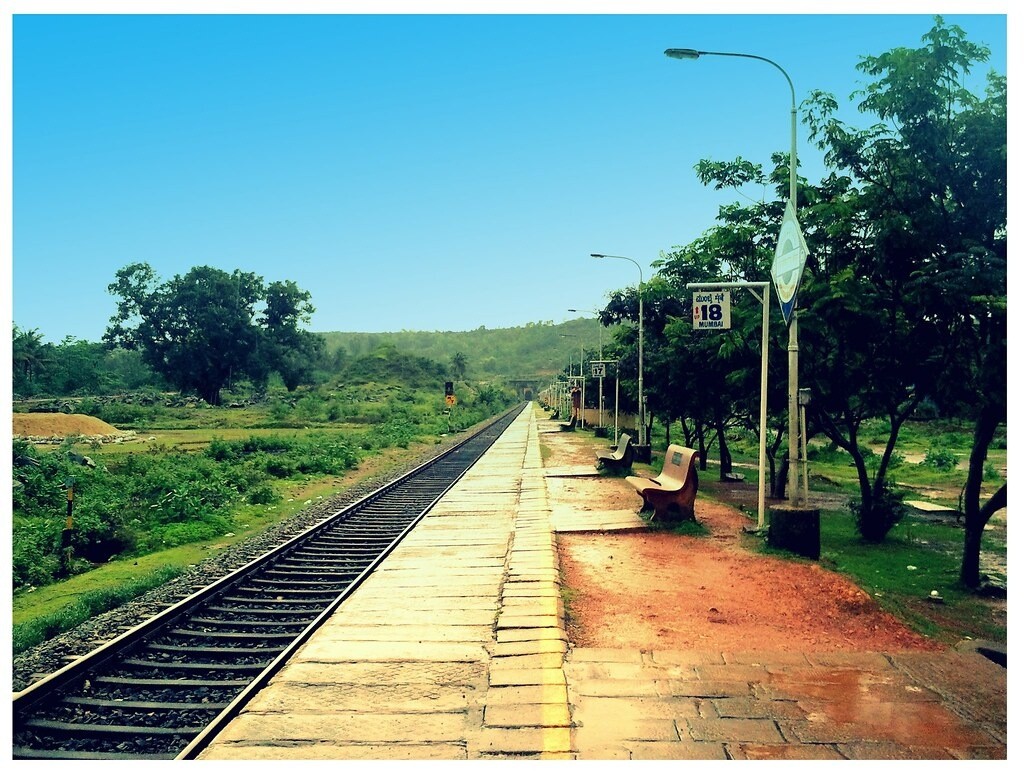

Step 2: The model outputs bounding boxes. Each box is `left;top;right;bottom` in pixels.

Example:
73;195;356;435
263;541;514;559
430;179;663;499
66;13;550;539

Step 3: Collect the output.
591;253;646;447
568;309;603;428
666;46;801;503
554;347;572;410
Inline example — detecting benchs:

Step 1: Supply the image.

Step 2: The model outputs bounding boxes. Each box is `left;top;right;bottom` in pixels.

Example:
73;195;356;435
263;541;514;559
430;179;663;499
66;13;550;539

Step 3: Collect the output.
551;409;559;419
624;443;703;528
559;416;577;431
544;405;550;411
595;432;633;474
